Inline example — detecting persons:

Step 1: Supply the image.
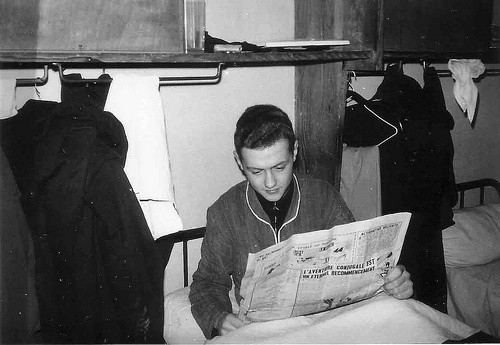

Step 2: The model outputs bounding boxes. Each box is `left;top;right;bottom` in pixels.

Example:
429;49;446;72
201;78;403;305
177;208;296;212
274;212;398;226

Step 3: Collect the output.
189;104;414;339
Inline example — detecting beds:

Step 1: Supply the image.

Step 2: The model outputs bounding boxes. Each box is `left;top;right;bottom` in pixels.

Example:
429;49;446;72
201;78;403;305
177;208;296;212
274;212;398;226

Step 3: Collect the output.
441;177;500;339
151;225;500;343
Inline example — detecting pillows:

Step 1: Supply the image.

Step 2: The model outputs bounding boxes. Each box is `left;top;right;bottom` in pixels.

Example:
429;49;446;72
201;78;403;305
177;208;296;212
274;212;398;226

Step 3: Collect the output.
442;203;500;269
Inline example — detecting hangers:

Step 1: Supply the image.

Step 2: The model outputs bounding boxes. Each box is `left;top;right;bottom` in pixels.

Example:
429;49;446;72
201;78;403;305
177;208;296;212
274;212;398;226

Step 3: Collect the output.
346;68;398;148
34;75;45;100
